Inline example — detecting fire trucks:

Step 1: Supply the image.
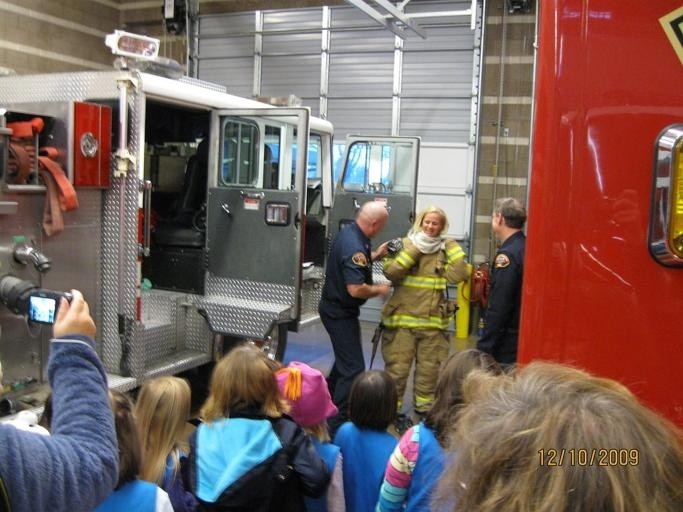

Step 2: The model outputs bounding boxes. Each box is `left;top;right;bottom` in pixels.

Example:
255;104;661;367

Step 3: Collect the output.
0;28;421;433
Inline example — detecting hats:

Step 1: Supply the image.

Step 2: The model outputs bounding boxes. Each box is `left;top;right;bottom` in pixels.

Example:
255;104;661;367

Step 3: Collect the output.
276;361;340;427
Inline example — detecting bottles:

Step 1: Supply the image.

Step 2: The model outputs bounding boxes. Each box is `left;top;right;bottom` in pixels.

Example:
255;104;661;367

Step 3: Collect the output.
140;279;152;321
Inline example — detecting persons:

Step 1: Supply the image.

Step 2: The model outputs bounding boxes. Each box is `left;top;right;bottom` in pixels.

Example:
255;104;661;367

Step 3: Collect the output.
40;390;176;512
317;201;399;411
185;340;332;511
271;352;351;511
371;204;469;420
428;360;683;512
479;195;529;373
125;376;198;494
0;286;121;512
372;348;504;512
333;367;403;511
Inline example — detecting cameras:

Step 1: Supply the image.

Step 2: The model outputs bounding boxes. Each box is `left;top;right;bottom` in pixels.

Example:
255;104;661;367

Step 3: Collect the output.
388;239;403;254
28;290;72;326
